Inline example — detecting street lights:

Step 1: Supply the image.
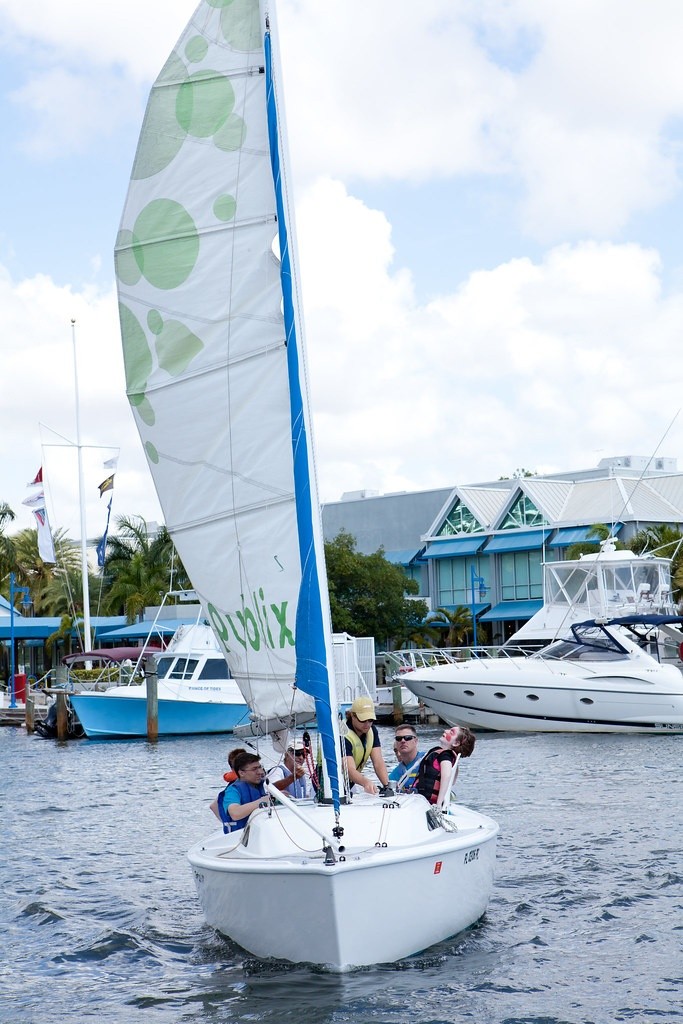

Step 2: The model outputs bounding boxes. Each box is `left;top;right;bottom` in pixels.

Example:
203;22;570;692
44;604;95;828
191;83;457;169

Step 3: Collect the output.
471;577;490;656
10;587;34;708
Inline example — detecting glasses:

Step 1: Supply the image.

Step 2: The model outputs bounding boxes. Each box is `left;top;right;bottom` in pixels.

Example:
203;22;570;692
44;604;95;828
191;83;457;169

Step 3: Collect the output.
288;750;305;759
393;748;398;753
394;734;418;742
352;713;374;723
241;765;262;771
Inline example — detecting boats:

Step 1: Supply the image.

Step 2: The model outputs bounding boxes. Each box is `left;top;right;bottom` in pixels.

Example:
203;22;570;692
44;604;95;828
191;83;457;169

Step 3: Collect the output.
379;614;683;734
68;588;378;738
494;537;683;672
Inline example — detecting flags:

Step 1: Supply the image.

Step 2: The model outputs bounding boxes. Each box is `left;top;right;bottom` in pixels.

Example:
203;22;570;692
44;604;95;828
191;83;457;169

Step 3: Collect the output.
103;456;118;468
27;467;44;488
98;474;113;498
22;490;44;507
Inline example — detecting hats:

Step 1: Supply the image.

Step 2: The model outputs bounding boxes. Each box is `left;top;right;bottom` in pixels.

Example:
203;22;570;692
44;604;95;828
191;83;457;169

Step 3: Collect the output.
351;696;377;721
287;738;311;754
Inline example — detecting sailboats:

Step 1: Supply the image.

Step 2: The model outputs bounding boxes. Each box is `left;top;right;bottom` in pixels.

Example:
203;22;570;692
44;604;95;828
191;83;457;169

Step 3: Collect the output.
113;2;500;974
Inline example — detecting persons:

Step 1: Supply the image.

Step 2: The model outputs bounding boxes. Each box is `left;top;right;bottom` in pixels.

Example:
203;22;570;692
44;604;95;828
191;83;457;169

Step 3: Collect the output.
412;726;476;808
209;752;270;834
312;697;390;798
388;724;428;793
265;739;311;798
224;748;289;797
